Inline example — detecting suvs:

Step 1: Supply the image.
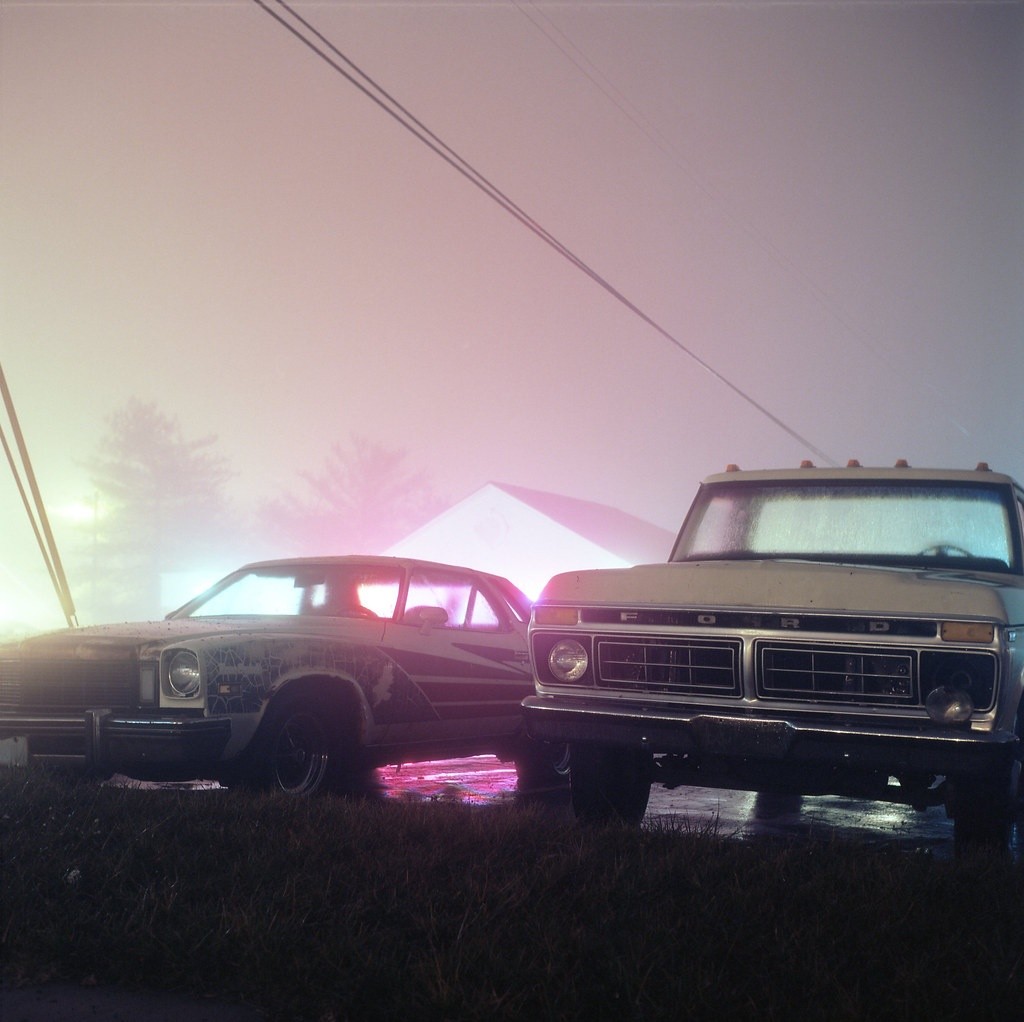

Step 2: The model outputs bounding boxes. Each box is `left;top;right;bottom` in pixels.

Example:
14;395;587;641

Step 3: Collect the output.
519;460;1024;845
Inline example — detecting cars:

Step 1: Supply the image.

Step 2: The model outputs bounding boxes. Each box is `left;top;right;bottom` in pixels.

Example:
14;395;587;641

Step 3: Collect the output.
0;554;541;795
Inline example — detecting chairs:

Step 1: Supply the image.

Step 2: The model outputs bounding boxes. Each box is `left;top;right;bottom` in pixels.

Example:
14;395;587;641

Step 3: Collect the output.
402;605;449;627
